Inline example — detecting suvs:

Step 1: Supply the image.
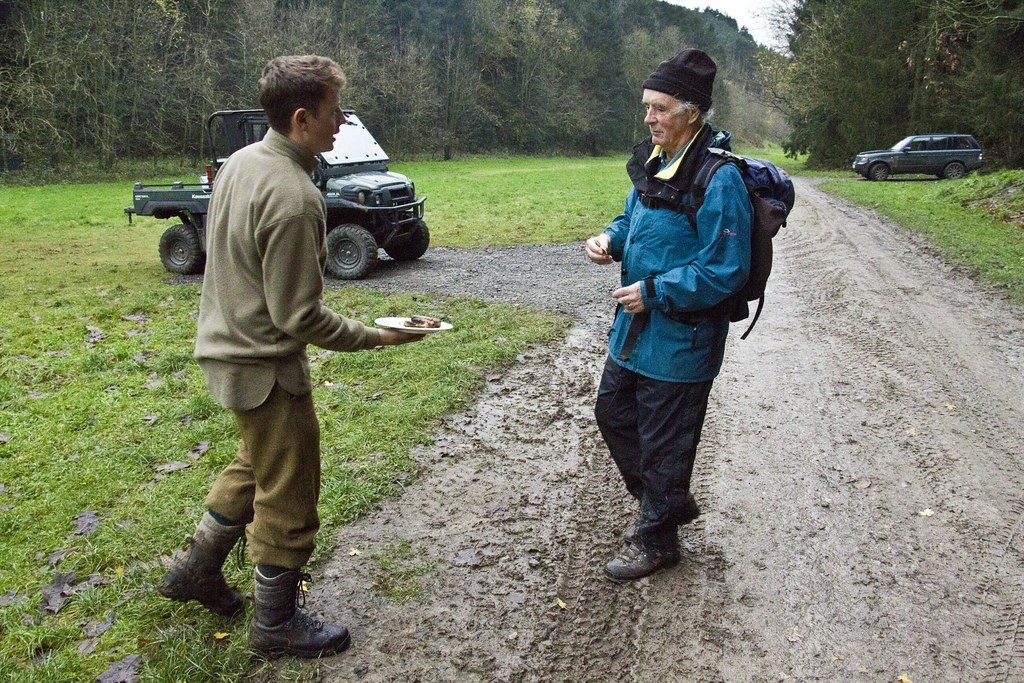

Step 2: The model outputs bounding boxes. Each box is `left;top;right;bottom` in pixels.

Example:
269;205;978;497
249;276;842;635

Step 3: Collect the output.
121;105;430;280
850;133;983;183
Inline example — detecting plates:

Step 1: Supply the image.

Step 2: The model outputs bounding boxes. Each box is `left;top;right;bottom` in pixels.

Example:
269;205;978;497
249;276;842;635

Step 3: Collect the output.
373;316;453;334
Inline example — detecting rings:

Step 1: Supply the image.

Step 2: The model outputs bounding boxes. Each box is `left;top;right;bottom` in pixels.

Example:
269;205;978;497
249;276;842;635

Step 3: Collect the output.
628;304;631;309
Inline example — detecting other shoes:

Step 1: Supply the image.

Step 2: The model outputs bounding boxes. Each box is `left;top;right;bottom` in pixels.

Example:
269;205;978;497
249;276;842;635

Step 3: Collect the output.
604;546;677;579
623;496;701;544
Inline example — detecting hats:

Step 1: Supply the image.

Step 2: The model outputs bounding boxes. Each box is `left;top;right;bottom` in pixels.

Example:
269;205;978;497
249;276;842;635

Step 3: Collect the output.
641;48;717;113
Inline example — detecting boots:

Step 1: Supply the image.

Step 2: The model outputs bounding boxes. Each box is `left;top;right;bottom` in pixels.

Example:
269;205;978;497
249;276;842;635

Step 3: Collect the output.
156;509;248;617
246;566;352;658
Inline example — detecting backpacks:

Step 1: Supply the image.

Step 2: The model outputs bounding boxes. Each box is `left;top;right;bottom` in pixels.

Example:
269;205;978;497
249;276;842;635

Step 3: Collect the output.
616;149;795;361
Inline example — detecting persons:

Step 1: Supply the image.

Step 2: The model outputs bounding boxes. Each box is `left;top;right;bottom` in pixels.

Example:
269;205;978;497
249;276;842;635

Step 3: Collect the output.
156;56;427;658
586;50;755;580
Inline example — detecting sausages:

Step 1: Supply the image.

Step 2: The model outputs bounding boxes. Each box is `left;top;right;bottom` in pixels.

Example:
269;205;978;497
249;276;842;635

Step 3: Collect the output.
404;315;441;327
